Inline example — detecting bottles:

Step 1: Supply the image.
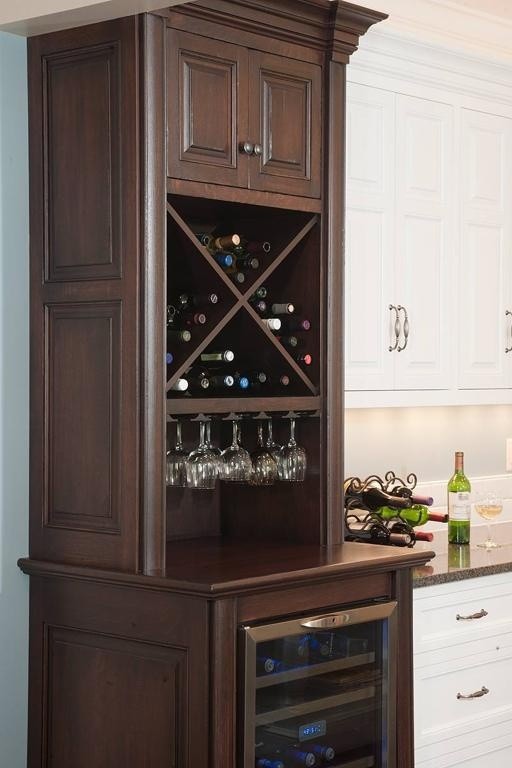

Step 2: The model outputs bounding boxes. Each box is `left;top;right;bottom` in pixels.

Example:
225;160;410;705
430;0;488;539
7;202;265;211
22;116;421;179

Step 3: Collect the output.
169;345;293;397
254;741;337;767
249;286;315;369
339;478;450;545
192;228;272;285
254;638;331;674
166;290;219;366
445;450;473;545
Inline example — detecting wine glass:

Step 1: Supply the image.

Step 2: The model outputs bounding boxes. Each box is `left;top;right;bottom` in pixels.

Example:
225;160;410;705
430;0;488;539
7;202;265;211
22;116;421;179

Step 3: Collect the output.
475;487;504;549
164;415;308;491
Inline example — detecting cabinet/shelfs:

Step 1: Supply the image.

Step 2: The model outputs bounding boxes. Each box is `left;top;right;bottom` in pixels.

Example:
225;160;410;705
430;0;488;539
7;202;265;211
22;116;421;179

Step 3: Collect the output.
347;79;457;391
16;12;436;766
412;571;511;767
456;105;512;391
